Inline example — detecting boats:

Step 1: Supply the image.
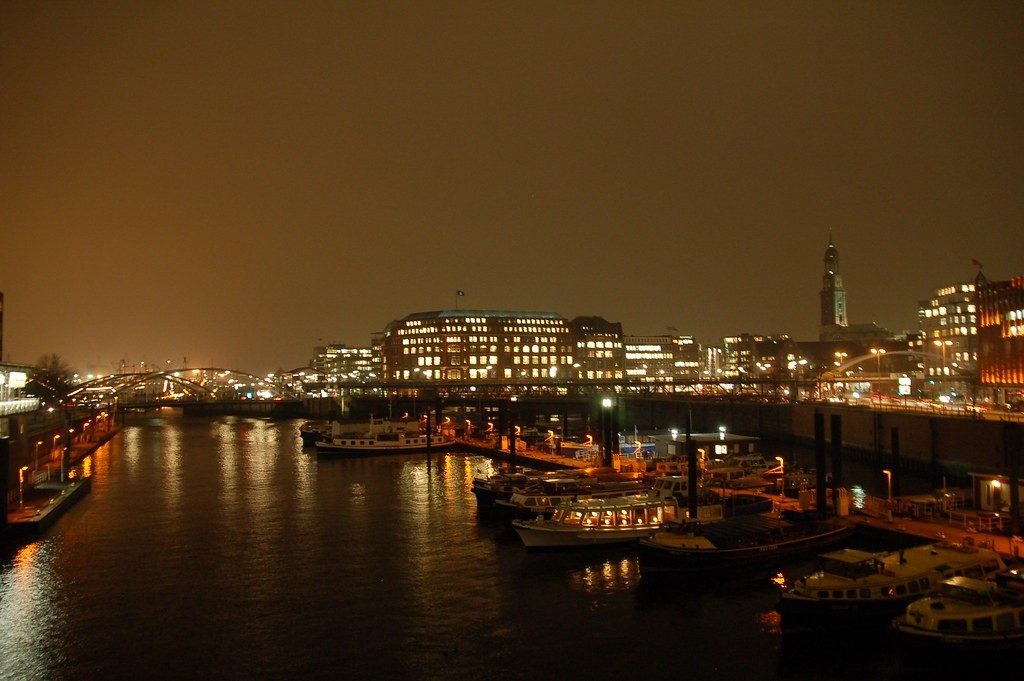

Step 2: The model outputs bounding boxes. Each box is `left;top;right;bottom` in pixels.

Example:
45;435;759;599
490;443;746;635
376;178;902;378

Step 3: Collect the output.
771;536;1010;632
471;464;729;553
634;505;857;581
299;411;455;456
887;563;1024;659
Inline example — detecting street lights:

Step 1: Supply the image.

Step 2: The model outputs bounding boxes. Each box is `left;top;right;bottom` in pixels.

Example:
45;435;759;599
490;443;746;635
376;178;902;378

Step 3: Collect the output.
775;455;786;504
18;466;29;512
882;468;893;523
466;419;471;441
547;429;554;459
444;417;451;442
60;446;69;482
635;440;642;471
35;441;44;471
83;423;90;441
67;428;74;451
586;434;593;465
869;348;888;409
53;434;61;462
835;349;848;363
697;448;706;485
933;339;954;408
514;425;521;452
